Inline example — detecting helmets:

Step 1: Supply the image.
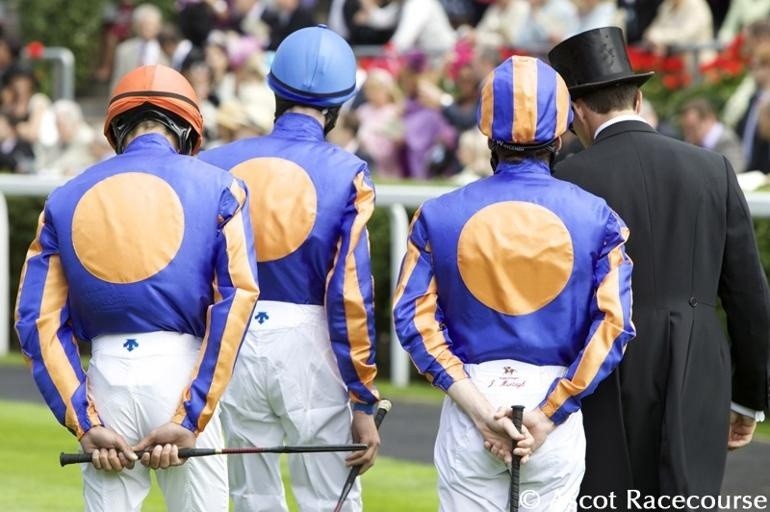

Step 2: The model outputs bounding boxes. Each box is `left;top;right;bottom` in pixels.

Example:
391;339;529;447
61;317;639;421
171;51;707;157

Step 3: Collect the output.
266;22;361;109
102;61;205;158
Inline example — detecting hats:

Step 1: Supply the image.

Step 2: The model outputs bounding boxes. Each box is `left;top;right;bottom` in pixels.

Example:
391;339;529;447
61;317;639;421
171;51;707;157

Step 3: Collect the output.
547;26;657;92
473;53;576;152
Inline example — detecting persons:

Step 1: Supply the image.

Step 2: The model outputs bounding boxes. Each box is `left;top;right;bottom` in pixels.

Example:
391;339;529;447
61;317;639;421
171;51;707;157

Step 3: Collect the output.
388;50;637;512
1;0;770;190
195;21;387;512
546;24;769;512
14;62;260;512
